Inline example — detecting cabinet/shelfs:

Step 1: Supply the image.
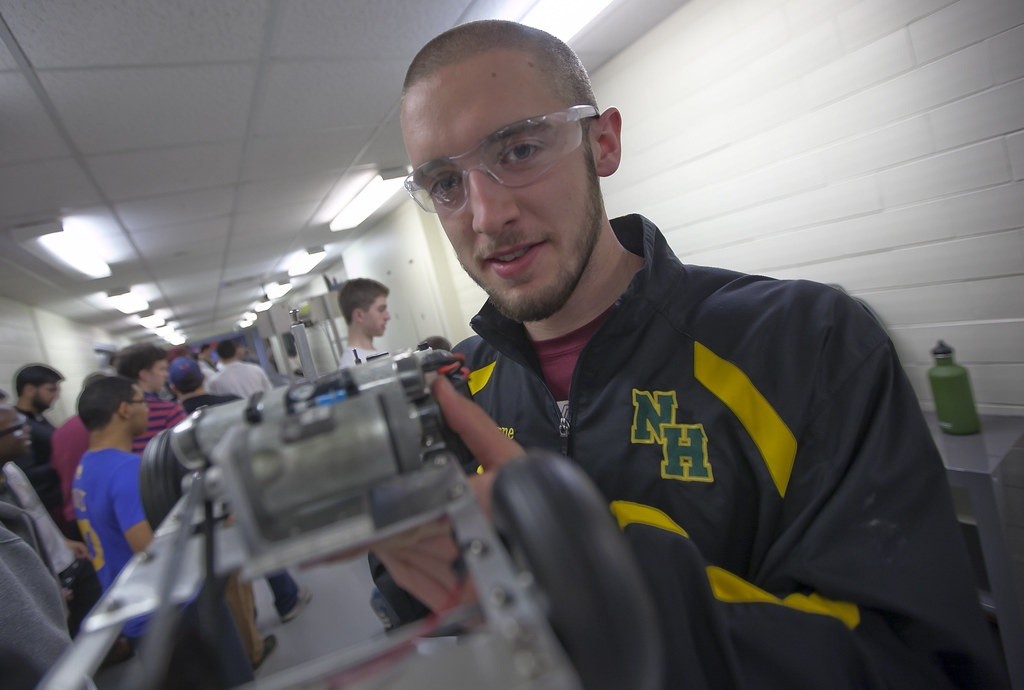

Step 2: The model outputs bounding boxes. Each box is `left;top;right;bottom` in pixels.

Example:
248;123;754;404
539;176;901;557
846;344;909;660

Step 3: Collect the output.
921;409;1024;690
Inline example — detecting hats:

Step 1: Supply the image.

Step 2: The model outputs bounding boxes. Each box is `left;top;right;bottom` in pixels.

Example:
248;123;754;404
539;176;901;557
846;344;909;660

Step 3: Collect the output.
167;357;203;393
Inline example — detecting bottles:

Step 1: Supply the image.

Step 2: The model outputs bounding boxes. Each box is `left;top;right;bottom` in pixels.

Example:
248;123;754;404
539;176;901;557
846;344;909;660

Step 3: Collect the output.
927;339;981;435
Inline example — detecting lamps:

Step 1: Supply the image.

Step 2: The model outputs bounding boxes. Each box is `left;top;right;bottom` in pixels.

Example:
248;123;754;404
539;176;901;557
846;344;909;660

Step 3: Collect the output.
14;222;112;282
106;286;150;316
330;166;413;232
288;247;327;278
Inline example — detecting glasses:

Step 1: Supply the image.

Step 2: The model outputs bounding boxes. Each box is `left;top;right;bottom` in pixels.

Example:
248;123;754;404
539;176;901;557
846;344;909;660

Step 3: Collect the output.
0;418;31;439
403;106;602;214
113;397;149;414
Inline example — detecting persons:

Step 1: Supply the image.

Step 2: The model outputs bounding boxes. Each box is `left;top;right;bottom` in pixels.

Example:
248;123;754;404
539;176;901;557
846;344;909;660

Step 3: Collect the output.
73;374;226;689
337;277;390;366
12;364;135;668
50;416;88;522
166;355;312;623
367;19;1009;690
0;405;88;643
168;341;272;397
117;346;276;671
0;502;97;690
